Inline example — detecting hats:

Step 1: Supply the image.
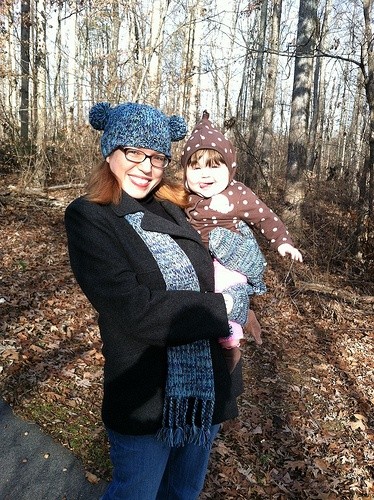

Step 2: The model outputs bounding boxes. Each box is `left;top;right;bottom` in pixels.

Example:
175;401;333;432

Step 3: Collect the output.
89;102;187;159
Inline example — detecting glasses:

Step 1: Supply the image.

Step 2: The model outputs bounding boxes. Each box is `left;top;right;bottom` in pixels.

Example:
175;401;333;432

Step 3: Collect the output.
119;146;170;168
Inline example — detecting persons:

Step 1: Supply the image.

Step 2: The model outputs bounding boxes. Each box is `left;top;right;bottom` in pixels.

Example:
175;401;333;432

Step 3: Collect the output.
64;102;245;500
181;109;304;375
